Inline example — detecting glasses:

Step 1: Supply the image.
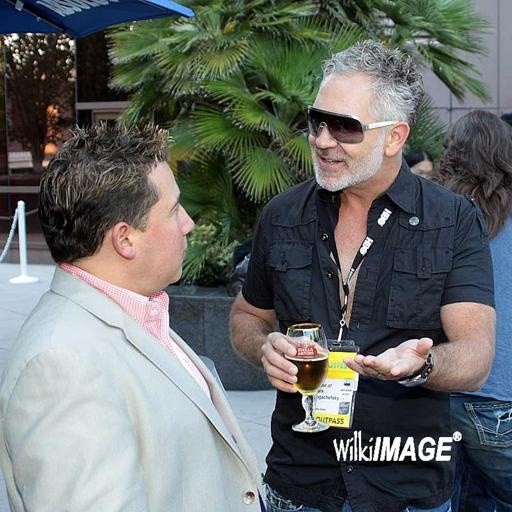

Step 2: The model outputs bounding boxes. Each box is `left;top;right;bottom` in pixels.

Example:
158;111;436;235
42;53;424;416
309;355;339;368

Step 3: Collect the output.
307;105;400;143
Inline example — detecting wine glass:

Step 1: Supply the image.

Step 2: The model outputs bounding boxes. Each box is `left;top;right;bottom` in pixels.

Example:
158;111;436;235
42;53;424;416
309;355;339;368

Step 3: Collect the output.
284;322;329;433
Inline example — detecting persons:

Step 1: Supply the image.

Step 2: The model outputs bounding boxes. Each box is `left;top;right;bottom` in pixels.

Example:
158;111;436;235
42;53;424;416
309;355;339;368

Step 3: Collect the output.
230;39;511;511
0;120;266;512
